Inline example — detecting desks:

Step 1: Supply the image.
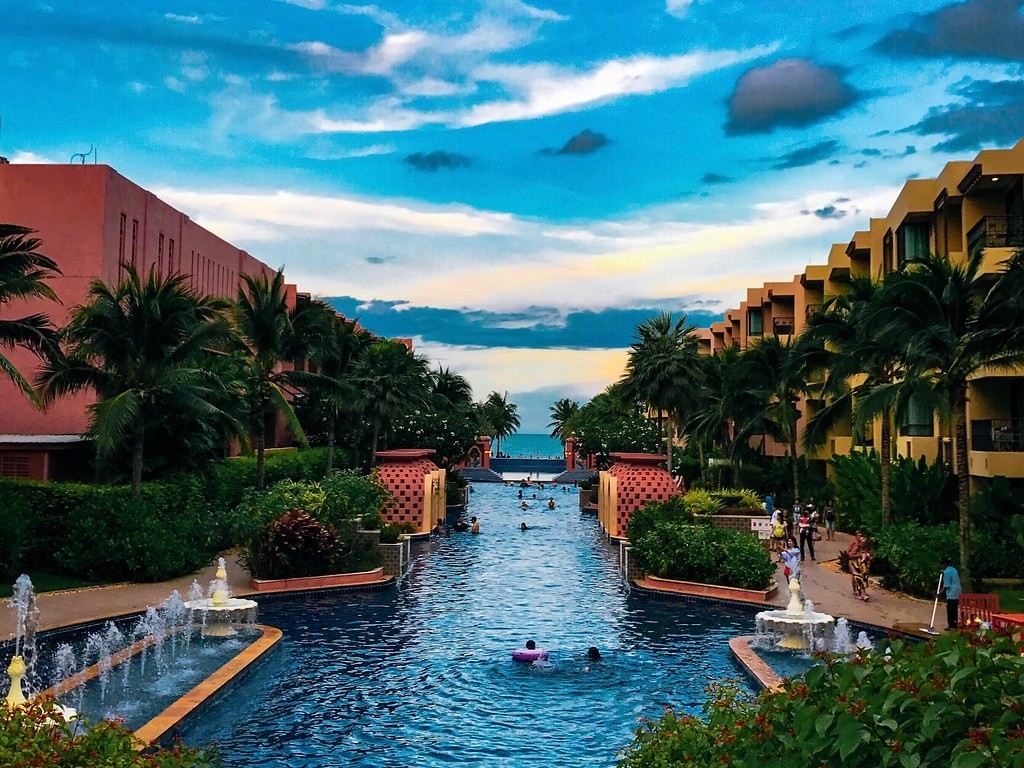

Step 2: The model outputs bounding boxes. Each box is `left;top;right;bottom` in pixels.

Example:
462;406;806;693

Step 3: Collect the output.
990;612;1024;655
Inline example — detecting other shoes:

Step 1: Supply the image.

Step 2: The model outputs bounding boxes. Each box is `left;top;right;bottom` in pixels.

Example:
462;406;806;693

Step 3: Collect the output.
945;626;956;631
800;557;804;561
826;537;836;541
811;557;816;561
770;548;777;552
859;595;869;602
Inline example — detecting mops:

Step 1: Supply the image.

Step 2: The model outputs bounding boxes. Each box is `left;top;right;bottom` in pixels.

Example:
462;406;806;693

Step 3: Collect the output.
919;574;943;636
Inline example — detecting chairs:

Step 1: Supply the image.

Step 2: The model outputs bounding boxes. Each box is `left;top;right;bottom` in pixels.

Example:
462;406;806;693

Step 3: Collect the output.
958;594;1000;623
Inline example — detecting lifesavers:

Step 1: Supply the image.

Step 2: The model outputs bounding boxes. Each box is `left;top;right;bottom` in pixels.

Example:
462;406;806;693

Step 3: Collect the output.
513;649;548;660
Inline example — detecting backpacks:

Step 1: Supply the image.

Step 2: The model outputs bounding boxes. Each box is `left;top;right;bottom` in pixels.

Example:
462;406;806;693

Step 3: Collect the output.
826;509;835;521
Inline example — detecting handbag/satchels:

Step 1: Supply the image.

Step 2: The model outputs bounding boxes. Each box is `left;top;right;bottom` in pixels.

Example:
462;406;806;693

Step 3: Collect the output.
784;566;792;577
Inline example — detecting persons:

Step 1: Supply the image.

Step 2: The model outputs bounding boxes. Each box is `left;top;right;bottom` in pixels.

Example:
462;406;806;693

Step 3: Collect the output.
761;492;838;563
504;476;570;509
933;556;961;631
452;517;468;532
521;522;526;528
525;640;536;650
847;530;875;602
587;647;600;657
776;536;805;603
469;486;475;493
470;517;479;532
